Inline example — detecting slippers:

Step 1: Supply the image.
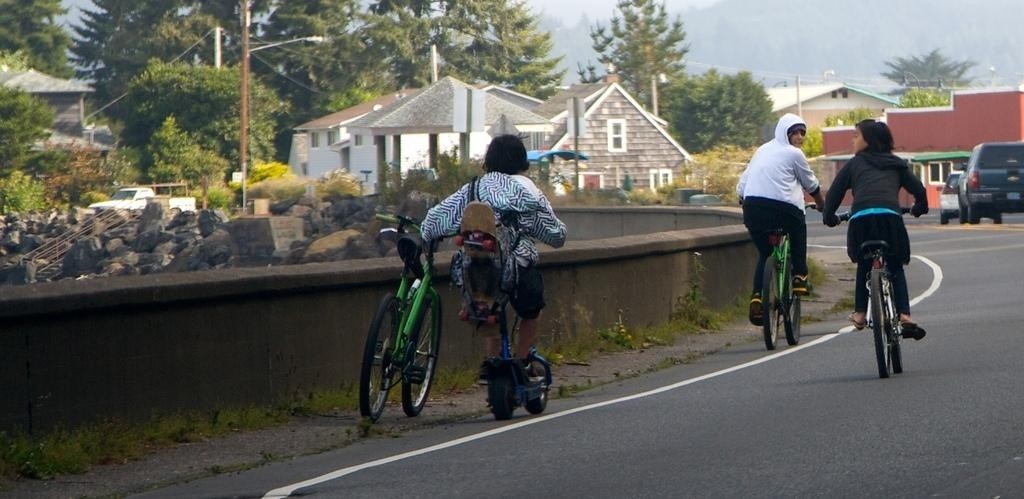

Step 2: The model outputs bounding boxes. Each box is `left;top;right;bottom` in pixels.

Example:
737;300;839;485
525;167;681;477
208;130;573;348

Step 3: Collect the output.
847;313;866;330
901;319;925;340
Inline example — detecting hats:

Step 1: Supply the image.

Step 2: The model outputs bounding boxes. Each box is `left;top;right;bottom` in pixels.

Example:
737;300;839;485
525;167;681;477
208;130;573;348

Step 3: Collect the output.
789;124;806;133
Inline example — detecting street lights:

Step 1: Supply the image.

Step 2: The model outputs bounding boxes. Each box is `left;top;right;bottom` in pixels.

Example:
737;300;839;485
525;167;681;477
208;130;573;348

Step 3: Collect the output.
240;34;326;213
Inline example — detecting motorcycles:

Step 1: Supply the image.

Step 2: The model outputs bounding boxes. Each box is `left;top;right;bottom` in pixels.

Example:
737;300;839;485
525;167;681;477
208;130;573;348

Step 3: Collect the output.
484;292;553;420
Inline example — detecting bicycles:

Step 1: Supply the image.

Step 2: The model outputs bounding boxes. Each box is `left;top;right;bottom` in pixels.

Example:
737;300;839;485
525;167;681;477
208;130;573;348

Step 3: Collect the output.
357;213;455;423
739;201;816;350
833;208;914;378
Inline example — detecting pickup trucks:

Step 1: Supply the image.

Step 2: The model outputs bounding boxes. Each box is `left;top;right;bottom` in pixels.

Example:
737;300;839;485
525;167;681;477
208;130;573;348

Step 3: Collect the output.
88;186;197;217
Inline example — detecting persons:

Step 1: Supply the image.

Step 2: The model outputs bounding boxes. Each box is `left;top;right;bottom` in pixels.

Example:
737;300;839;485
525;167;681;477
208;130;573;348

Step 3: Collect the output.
821;120;925;339
421;135;568;381
736;113;827;327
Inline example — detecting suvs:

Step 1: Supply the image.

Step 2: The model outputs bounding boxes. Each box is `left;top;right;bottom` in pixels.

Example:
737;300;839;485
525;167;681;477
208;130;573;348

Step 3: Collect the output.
958;141;1024;225
936;170;1004;225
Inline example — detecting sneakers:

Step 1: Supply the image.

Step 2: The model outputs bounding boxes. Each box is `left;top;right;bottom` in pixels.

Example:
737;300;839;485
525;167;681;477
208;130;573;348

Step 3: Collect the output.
523;364;544;383
792;274;809;296
478;360;490;384
749;295;762;326
